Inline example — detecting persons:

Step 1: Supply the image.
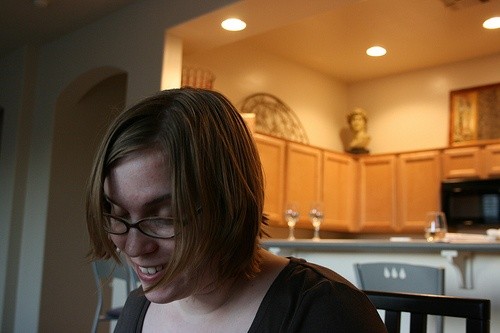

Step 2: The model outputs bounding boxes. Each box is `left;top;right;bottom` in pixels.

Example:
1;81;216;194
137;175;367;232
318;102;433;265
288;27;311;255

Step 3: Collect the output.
86;87;387;333
347;108;375;151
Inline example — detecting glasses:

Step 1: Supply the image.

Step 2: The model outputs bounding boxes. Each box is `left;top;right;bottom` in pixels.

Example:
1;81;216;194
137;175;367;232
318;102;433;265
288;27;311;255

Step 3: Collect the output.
97;205;202;239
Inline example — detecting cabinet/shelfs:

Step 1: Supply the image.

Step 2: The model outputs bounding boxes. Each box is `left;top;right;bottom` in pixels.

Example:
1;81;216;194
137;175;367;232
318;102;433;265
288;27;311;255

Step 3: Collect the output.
255;129;500;232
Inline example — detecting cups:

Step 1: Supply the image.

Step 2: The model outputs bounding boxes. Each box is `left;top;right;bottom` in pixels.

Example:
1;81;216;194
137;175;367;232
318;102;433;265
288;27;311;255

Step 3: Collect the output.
424;212;447;241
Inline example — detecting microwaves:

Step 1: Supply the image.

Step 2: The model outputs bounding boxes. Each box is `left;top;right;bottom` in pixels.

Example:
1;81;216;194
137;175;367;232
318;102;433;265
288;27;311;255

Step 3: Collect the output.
440;178;500;228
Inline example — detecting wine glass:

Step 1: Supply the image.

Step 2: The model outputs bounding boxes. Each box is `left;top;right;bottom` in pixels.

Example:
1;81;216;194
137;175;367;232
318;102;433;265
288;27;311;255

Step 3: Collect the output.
309;204;323;240
284;202;300;240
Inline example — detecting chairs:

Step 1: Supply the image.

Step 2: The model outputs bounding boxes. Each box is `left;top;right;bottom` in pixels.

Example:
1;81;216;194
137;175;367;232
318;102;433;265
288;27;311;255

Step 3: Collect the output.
354;263;445;333
92;255;141;333
363;290;490;333
